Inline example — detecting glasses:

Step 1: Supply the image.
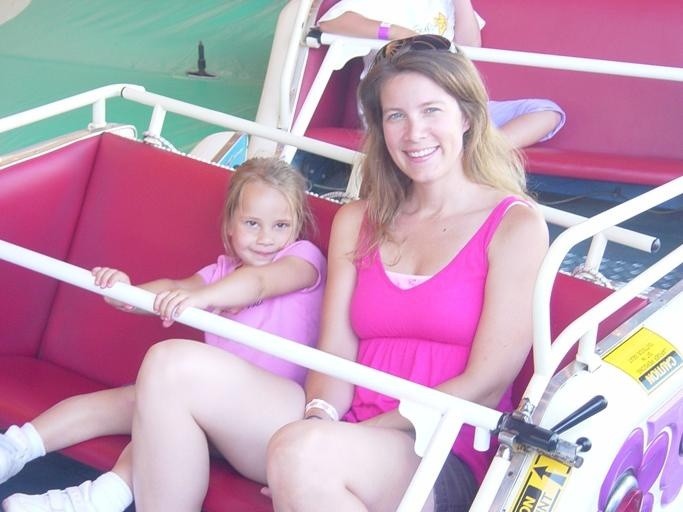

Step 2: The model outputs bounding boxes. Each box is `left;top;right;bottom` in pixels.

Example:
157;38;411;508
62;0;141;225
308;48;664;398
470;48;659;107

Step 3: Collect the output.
373;35;458;65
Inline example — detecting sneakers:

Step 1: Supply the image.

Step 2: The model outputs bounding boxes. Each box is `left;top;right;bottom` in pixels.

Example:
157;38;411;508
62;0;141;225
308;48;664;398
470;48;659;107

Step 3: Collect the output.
2;479;94;511
0;424;29;485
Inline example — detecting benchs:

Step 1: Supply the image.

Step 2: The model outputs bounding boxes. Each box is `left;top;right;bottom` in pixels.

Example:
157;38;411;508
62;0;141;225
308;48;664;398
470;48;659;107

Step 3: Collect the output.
0;133;647;512
291;1;682;186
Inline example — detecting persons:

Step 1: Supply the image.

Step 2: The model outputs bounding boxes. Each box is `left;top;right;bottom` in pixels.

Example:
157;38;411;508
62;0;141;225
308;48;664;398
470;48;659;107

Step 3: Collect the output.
318;1;567;157
130;34;552;512
0;156;327;512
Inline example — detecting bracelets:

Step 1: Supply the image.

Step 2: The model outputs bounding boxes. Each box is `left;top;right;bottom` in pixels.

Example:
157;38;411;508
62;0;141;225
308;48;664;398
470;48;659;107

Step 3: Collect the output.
304;397;341;424
378;21;391;40
